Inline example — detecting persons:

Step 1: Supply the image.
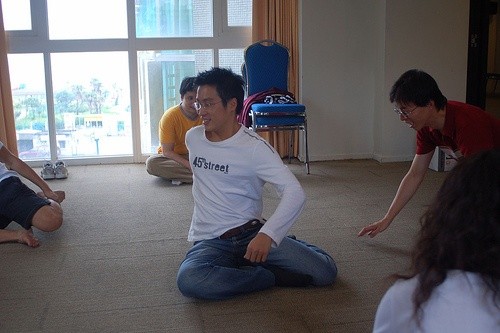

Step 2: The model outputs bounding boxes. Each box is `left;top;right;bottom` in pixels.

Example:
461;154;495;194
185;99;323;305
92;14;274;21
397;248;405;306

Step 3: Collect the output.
175;65;340;301
0;139;65;247
357;68;500;239
372;147;500;333
144;76;204;185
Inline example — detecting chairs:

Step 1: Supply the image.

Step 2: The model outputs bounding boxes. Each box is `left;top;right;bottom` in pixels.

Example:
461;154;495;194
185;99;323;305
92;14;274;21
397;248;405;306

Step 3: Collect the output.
242;39;310;174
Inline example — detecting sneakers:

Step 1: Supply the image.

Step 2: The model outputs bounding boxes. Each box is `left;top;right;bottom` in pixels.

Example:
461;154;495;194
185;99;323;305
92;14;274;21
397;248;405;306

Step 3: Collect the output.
41;161;68;179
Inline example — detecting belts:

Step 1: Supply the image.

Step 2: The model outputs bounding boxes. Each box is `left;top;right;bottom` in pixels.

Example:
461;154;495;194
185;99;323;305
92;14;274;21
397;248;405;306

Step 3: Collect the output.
218;219;260;240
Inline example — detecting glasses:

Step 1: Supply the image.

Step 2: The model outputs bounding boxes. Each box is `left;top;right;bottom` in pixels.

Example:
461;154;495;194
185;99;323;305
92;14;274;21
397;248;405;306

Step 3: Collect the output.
194;101;221;111
393;105;419;119
